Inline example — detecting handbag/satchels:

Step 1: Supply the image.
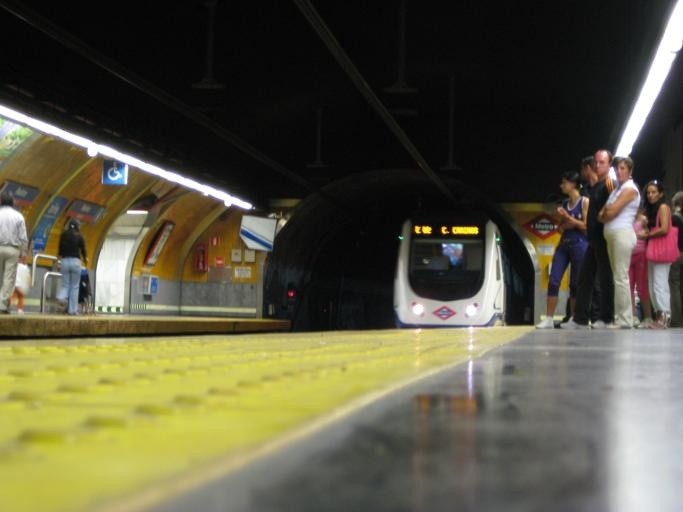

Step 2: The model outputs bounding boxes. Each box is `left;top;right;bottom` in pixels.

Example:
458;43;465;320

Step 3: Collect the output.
647;227;680;262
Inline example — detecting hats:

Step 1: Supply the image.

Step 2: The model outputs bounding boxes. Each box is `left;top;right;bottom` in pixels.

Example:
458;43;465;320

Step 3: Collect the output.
69;219;80;228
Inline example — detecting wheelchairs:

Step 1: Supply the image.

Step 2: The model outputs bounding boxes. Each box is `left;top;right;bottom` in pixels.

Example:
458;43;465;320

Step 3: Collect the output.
78;261;94;315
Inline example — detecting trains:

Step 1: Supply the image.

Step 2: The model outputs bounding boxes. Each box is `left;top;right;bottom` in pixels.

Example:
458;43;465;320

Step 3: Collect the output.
393;216;533;328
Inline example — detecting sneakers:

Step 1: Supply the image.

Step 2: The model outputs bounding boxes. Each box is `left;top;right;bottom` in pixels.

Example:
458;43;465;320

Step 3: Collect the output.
537;313;671;330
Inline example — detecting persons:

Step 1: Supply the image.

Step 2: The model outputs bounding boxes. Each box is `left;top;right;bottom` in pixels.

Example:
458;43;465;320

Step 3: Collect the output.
0;192;29;314
535;149;683;329
8;254;29;313
57;219;88;313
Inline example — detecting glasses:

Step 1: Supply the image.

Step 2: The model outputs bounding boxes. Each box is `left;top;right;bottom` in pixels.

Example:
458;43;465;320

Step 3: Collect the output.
648;180;659;186
